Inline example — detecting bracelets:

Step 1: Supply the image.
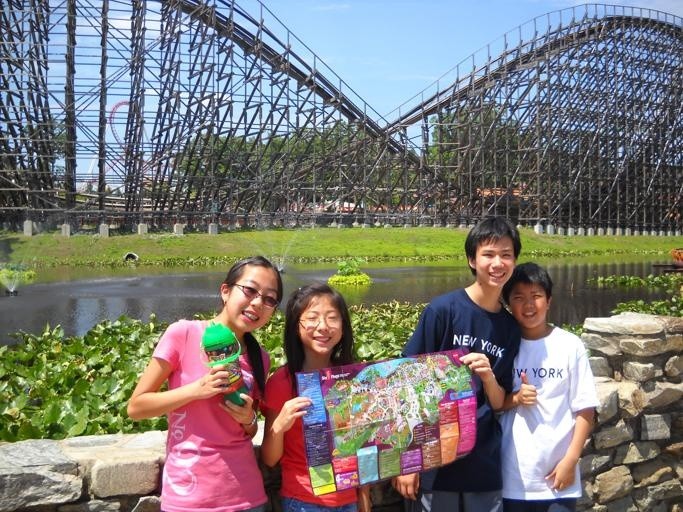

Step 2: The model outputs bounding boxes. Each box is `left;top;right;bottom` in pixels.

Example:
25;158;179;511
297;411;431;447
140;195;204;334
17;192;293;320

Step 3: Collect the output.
249;411;257;425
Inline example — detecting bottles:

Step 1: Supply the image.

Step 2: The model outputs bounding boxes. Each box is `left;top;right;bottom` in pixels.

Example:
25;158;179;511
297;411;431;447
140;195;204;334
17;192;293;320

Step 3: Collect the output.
200;321;248;406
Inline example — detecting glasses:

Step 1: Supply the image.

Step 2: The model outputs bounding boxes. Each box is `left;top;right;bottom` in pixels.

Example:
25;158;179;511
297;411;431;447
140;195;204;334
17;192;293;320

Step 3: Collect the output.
227;283;280;310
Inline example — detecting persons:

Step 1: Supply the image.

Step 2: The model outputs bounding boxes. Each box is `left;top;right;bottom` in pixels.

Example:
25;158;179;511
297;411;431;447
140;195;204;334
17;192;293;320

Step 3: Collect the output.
263;280;370;512
390;217;521;512
128;256;283;512
498;261;599;512
